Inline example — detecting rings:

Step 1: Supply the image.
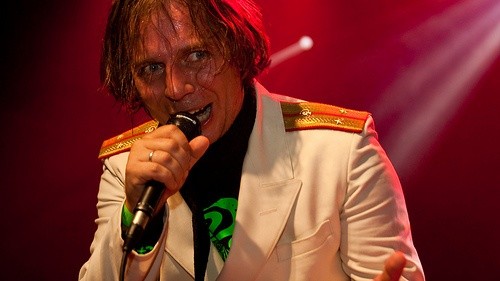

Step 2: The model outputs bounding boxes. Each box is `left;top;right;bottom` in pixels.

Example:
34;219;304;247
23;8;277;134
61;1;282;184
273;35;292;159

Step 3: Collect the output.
148;150;156;161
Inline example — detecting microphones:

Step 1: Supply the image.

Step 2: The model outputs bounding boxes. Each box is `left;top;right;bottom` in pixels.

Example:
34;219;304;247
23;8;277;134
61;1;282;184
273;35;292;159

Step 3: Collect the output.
125;113;201;251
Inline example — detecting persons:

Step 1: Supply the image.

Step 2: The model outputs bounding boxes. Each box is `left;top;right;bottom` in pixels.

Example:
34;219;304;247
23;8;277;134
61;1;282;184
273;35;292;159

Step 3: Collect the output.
78;0;427;281
